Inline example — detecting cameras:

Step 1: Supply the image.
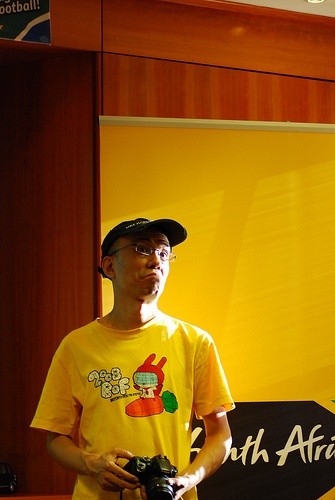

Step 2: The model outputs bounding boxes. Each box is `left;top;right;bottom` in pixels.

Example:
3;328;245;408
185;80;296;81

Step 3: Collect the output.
122;454;178;500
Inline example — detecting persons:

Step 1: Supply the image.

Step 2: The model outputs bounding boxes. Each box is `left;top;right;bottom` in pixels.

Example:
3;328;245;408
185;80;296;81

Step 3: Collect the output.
30;218;235;500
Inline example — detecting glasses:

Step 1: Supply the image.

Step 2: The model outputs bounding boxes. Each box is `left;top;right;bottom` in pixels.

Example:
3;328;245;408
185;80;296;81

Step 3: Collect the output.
106;243;176;263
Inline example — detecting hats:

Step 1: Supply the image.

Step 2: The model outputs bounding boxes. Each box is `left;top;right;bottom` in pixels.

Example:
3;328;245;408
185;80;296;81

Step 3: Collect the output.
97;218;187;281
